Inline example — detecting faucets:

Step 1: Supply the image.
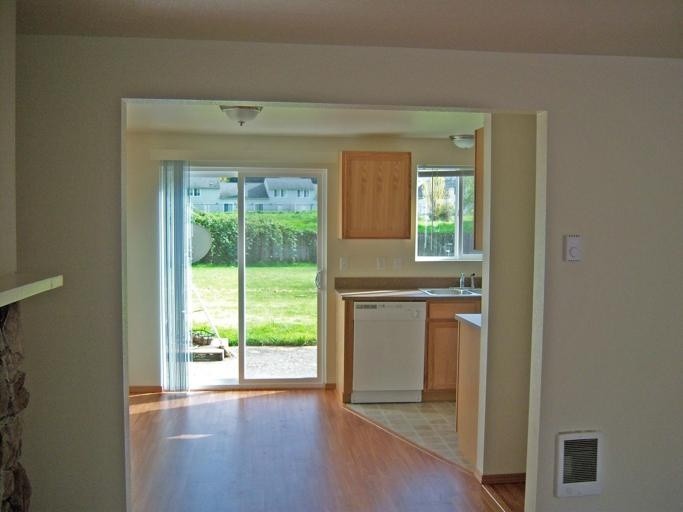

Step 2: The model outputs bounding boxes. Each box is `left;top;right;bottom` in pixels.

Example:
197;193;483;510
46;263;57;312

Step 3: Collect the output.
459;273;465;287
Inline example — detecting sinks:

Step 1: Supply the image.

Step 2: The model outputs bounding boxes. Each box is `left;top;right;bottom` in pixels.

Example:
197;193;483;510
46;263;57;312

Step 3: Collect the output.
417;287;477;296
470;288;482;296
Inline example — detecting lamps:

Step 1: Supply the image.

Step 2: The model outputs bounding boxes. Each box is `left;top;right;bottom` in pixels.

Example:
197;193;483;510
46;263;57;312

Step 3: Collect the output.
219;106;263;126
449;134;476;151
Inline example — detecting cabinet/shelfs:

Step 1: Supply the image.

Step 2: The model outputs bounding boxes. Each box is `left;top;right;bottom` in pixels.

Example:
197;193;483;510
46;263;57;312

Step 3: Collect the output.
337;151;412;240
474;127;484;251
422;298;481;403
454;321;481;467
